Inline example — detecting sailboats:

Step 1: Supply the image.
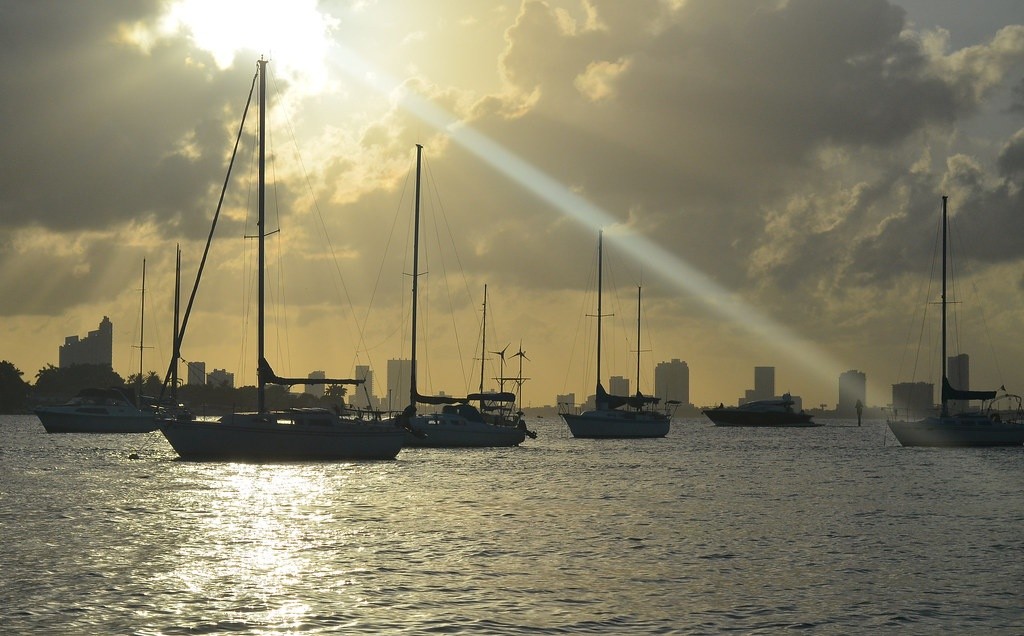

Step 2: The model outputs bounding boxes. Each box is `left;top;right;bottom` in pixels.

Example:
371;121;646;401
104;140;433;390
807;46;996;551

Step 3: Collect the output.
884;196;1024;447
31;55;538;464
559;229;672;439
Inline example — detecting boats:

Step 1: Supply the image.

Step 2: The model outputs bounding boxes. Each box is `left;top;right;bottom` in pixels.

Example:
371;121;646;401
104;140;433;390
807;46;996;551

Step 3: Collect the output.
704;392;823;427
